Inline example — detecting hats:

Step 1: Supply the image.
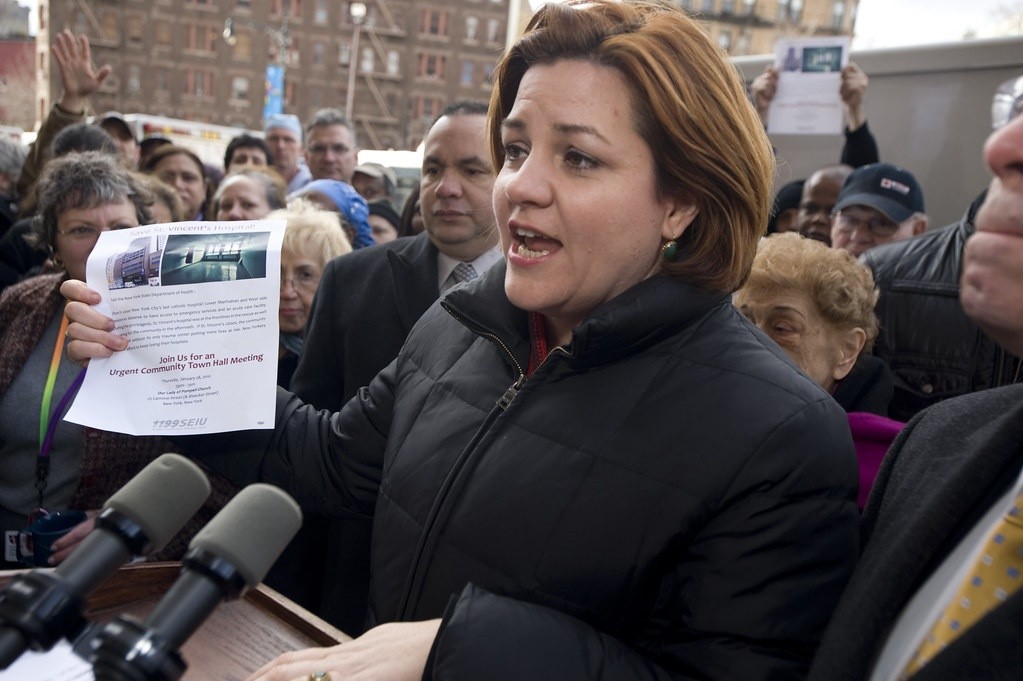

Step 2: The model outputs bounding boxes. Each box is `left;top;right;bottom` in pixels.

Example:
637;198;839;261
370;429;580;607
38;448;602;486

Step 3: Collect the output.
831;163;925;223
138;133;174;147
355;161;397;188
262;111;302;140
91;110;138;146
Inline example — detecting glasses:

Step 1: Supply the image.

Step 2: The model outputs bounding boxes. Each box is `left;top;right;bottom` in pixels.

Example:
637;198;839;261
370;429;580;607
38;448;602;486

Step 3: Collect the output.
55;224;134;240
280;270;318;290
829;213;902;236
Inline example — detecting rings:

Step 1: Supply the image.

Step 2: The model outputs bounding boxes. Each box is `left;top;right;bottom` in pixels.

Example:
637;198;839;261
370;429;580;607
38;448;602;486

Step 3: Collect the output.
307;670;331;681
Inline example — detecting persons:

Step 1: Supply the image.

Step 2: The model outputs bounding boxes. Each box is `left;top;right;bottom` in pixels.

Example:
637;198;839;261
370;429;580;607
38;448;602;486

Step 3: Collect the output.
747;60;881;242
289;99;504;419
1;28;428;297
793;72;1023;681
793;162;855;252
825;184;1023;429
56;1;865;681
254;194;356;396
730;230;910;516
0;151;164;569
826;162;931;265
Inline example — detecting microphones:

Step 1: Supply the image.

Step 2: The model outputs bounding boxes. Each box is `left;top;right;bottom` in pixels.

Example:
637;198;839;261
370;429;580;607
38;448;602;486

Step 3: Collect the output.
2;452;302;681
